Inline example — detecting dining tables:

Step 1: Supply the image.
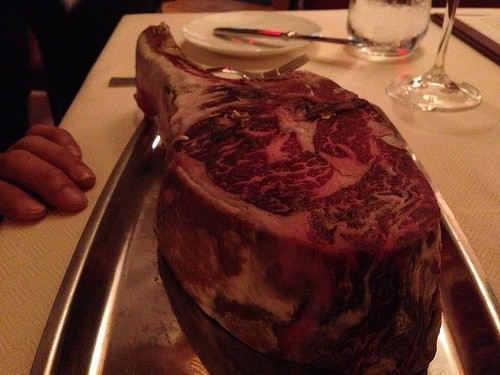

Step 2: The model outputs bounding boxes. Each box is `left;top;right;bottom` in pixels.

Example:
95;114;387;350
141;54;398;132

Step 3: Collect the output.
2;6;500;374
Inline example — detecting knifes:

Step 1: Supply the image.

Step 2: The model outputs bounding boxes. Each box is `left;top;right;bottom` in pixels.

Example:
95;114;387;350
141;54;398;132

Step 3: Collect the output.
215;24;363;46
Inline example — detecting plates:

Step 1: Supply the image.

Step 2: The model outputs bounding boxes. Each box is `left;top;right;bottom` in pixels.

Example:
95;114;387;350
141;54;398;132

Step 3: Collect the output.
183;11;320;58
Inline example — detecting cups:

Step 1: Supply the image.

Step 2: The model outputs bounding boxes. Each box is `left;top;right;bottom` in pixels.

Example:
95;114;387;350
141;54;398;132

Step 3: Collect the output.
346;2;433;61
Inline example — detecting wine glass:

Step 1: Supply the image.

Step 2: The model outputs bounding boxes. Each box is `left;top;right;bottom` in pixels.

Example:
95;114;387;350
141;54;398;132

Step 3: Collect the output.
386;0;481;110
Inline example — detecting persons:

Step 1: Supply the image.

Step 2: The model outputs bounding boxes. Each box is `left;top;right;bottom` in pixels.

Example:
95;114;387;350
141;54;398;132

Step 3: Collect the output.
0;0;119;222
